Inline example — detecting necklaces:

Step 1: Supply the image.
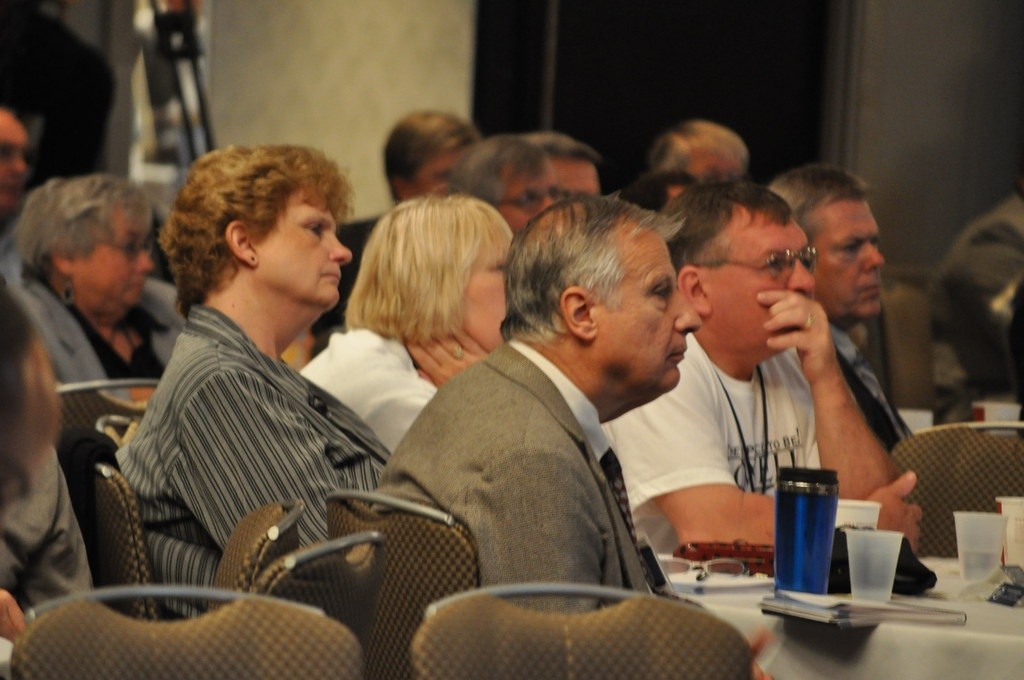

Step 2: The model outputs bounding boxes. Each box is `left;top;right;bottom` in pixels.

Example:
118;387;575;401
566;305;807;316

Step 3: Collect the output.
119;325;137;350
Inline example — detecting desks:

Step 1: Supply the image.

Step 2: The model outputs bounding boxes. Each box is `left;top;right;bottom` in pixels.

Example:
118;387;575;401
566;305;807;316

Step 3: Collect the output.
668;554;1024;680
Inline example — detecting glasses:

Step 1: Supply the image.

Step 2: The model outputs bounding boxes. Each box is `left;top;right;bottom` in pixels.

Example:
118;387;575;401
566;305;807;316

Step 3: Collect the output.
491;188;546;214
100;237;153;260
694;248;818;282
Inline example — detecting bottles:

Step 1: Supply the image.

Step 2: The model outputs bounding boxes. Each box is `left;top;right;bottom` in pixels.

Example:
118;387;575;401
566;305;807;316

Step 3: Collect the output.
774;467;838;595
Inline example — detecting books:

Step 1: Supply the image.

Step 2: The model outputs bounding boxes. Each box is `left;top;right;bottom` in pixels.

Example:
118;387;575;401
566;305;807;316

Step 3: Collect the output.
759;588;968;628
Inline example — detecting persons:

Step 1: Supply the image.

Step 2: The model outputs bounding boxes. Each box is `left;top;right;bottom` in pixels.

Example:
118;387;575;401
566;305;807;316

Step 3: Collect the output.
369;190;778;680
0;273;91;641
112;142;395;624
0;0;1023;458
602;178;925;571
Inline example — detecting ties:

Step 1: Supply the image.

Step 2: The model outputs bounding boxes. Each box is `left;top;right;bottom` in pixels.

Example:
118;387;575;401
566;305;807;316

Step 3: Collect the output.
600;447;656;595
852;351;906;441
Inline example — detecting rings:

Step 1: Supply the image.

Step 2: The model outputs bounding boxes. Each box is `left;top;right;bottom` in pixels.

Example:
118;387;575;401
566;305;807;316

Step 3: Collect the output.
804;312;815;327
454;346;463;359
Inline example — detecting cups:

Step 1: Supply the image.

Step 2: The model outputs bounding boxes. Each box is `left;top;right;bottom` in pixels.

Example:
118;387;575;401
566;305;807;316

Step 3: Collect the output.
834;497;881;531
972;399;1022;422
953;510;1011;587
900;408;933;432
994;496;1024;568
845;529;904;603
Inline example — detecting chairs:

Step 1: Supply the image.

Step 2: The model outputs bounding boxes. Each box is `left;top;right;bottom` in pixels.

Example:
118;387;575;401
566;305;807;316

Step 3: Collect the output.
0;281;1024;680
